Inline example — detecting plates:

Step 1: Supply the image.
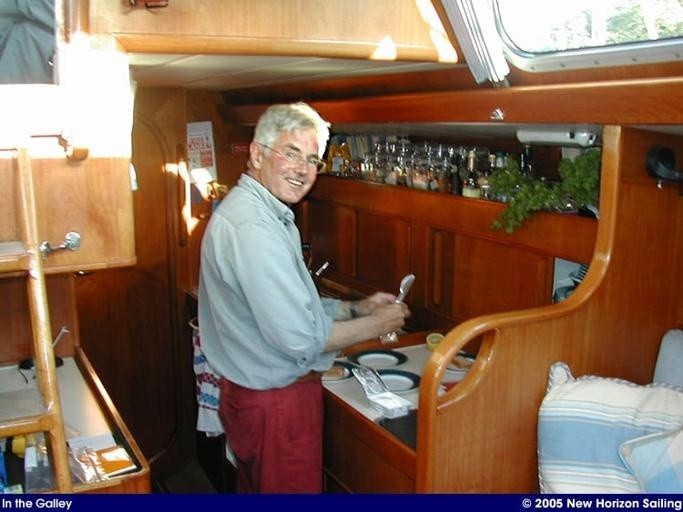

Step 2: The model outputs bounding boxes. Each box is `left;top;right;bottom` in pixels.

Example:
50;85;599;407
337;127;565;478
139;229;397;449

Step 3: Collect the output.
320;359;360;381
381;369;421;394
347;347;409;371
442;353;478;375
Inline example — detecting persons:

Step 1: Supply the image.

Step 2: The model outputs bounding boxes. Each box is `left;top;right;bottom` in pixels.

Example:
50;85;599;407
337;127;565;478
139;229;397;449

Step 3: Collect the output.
197;97;412;494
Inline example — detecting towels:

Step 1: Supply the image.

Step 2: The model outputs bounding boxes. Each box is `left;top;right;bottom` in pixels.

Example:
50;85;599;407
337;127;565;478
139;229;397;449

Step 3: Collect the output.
192;327;227;437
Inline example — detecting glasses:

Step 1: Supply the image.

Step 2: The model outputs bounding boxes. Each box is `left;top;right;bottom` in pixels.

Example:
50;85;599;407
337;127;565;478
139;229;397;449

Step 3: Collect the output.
254;139;326;173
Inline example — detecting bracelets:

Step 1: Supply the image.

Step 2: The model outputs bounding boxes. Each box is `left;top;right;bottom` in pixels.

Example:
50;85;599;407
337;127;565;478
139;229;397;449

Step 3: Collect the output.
349;299;359;318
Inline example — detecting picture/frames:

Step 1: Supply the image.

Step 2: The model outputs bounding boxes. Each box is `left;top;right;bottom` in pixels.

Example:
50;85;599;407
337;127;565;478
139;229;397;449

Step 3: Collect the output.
0;0;90;85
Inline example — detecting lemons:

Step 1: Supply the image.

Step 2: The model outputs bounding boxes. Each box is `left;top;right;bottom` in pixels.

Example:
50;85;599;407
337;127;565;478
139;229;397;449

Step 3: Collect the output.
425;333;445;350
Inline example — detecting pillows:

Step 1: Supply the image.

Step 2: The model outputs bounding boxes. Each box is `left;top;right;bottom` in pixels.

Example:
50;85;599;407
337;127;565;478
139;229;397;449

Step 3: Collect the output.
537;361;683;494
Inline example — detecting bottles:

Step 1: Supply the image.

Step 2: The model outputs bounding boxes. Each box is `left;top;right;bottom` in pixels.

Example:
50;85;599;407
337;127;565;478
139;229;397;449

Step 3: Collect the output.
321;134;535;210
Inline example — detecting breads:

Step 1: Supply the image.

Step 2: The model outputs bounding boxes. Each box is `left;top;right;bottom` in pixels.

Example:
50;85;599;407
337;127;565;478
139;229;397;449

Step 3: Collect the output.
452;355;474;368
323;366;344;378
